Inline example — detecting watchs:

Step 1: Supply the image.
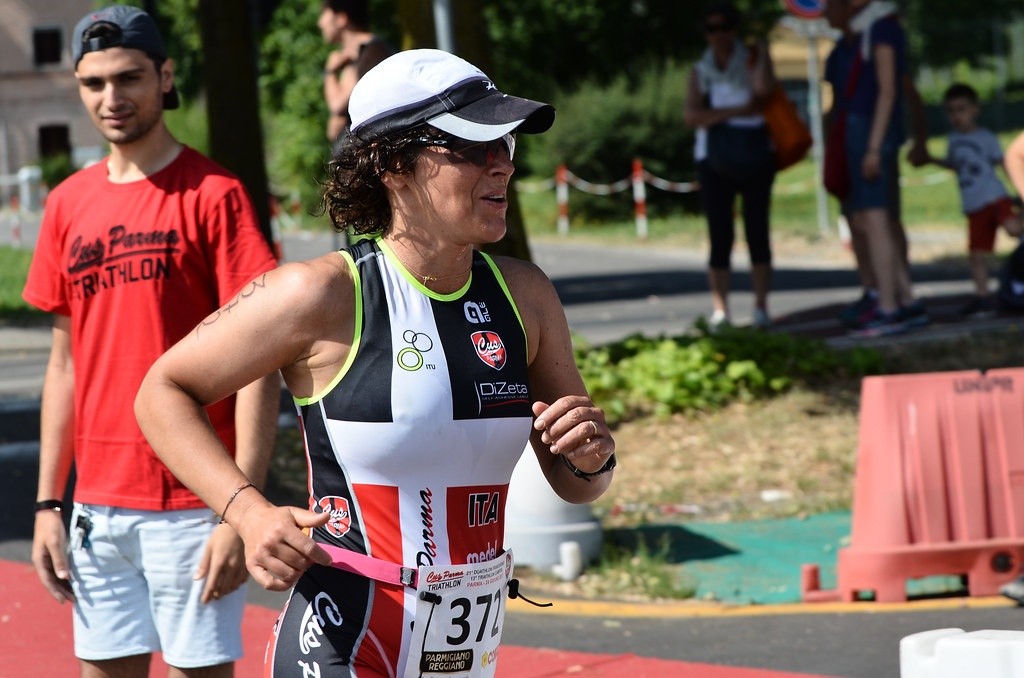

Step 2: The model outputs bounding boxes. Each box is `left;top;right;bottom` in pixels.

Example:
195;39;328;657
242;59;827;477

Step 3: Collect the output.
558;451;616;482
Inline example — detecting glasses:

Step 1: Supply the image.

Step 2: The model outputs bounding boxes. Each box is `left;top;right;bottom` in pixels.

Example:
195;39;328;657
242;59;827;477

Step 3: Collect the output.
414;131;517;167
704;20;731;32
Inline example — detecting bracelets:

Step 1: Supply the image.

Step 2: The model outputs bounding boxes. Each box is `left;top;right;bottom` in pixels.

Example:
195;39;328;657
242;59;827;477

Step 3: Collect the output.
219;483;260;523
34;499;64;512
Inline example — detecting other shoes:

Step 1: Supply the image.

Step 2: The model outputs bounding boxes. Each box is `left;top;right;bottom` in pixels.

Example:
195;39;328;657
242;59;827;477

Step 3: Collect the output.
709;309;727;326
849;308;908;338
751;308;769;329
839;288;877;320
900;305;928;325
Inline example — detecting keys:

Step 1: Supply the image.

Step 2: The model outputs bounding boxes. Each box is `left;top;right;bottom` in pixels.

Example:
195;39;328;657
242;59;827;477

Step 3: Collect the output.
71;515;92;549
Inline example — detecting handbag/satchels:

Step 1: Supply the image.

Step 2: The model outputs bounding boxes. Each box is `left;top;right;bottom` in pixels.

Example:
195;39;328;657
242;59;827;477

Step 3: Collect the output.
746;52;812;169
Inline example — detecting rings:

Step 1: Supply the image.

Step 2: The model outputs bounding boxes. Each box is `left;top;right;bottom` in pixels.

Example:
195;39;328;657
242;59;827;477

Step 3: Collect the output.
588;419;598;437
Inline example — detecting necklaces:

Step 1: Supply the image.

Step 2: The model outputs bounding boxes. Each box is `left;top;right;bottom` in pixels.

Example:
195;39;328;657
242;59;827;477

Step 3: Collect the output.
386;237;472;288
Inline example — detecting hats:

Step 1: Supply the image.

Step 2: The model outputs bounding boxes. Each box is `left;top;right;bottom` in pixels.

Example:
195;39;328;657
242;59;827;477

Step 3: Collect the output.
349;48;556;143
70;5;179;111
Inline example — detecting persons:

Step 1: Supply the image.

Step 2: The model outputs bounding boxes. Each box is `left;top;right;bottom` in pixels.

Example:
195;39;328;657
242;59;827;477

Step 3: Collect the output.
316;0;385;144
133;49;615;678
684;0;1024;333
21;4;280;678
